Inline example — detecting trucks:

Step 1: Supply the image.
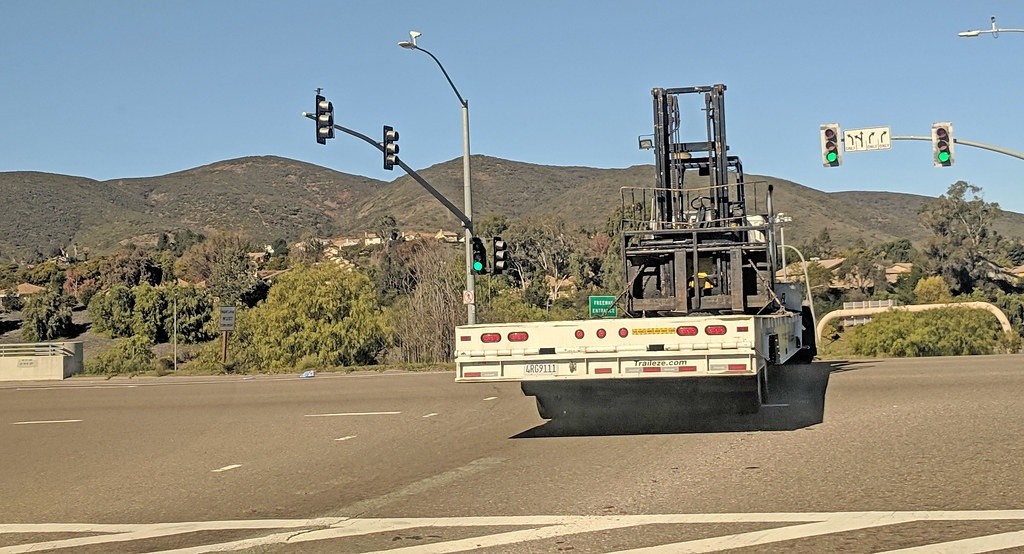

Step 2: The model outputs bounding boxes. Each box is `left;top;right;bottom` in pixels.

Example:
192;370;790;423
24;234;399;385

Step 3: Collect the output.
454;85;818;421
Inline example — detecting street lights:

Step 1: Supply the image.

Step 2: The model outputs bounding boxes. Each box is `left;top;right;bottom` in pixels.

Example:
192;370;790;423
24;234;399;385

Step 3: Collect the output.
398;31;478;327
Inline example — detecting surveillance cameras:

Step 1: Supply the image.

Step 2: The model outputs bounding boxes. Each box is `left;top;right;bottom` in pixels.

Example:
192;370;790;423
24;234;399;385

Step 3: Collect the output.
991;15;996;24
410;31;422;38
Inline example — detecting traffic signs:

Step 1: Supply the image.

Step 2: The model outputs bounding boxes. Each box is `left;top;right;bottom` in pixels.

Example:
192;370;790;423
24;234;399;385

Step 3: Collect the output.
844;127;891;152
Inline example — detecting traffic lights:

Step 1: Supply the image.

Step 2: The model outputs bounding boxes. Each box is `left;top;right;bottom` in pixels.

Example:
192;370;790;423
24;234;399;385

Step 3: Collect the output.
471;237;487;275
820;123;842;168
383;125;400;171
493;237;507;275
316;95;334;145
932;122;955;167
